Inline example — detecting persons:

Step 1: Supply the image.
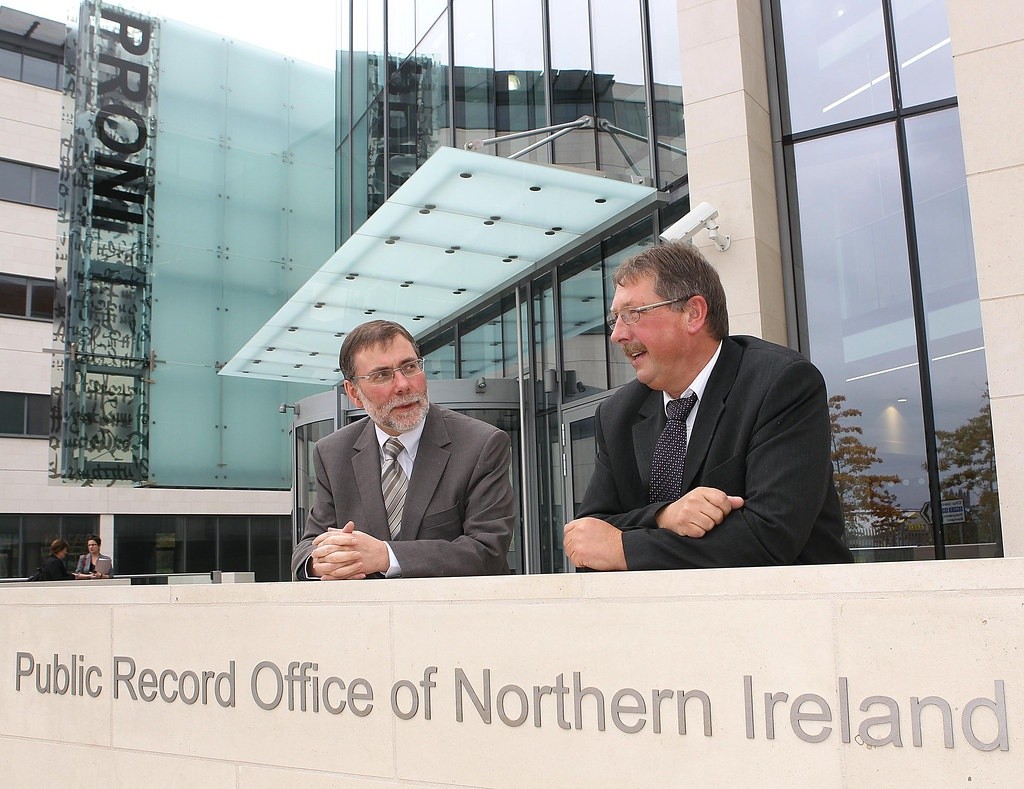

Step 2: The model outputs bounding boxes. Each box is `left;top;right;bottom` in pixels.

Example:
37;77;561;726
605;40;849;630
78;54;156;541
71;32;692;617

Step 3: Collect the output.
75;534;114;580
291;318;515;584
562;242;855;574
36;538;81;581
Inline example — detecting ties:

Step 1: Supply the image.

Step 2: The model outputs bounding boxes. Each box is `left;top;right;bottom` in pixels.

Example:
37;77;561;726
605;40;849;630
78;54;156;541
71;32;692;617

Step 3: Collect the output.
648;392;700;507
380;437;410;541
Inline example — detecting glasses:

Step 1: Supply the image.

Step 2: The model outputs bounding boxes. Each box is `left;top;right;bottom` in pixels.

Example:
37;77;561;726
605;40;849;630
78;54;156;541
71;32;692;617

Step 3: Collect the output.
351;356;426;386
607;292;701;332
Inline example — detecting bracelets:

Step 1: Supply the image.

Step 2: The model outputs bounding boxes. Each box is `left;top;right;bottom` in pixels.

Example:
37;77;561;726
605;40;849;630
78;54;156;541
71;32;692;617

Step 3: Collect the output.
100;573;103;579
